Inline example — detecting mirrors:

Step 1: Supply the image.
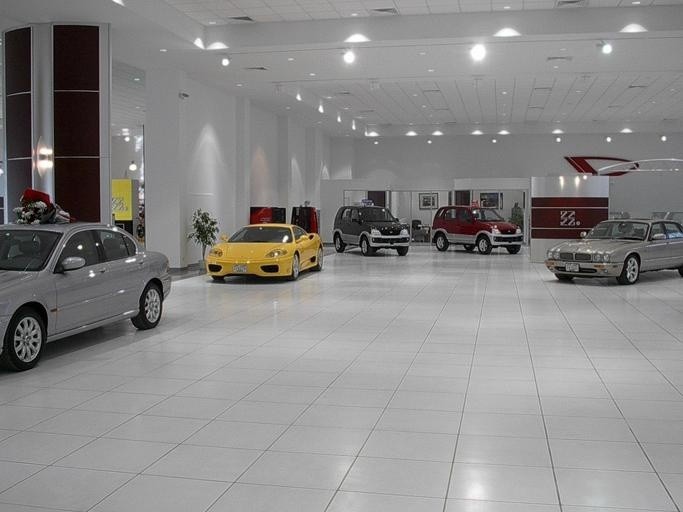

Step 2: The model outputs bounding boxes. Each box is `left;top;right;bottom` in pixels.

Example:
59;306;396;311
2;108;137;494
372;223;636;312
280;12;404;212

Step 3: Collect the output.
342;188;528;246
109;57;145;251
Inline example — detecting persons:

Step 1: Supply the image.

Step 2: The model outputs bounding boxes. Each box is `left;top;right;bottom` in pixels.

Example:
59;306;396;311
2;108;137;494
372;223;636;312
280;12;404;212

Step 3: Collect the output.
511;201;522;232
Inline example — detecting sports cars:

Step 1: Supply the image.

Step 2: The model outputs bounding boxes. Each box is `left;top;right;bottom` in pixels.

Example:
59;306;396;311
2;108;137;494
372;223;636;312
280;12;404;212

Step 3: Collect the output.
205;224;323;281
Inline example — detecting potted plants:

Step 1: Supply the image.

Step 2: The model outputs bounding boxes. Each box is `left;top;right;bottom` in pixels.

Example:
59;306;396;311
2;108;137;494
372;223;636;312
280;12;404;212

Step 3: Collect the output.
184;206;219;270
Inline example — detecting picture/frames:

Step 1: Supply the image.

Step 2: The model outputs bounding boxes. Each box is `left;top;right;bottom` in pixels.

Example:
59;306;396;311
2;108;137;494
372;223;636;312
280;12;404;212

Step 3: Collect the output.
418;192;438;210
479;192;503;209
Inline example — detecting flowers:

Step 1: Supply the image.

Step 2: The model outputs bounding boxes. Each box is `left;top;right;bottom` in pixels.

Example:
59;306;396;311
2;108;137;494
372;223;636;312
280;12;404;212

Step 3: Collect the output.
9;190;76;225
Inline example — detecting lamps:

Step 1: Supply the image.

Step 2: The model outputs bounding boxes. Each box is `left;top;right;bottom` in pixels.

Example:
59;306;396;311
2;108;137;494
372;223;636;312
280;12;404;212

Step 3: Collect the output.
36;137;54;178
124;160;136;179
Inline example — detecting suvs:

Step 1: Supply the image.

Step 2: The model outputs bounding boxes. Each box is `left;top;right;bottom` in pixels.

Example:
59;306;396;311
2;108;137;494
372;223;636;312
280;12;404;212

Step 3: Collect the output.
331;203;410;256
430;204;523;254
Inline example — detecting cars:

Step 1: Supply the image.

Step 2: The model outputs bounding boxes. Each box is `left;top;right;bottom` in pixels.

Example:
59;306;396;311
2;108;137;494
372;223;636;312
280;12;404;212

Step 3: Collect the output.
543;220;683;284
0;222;170;371
